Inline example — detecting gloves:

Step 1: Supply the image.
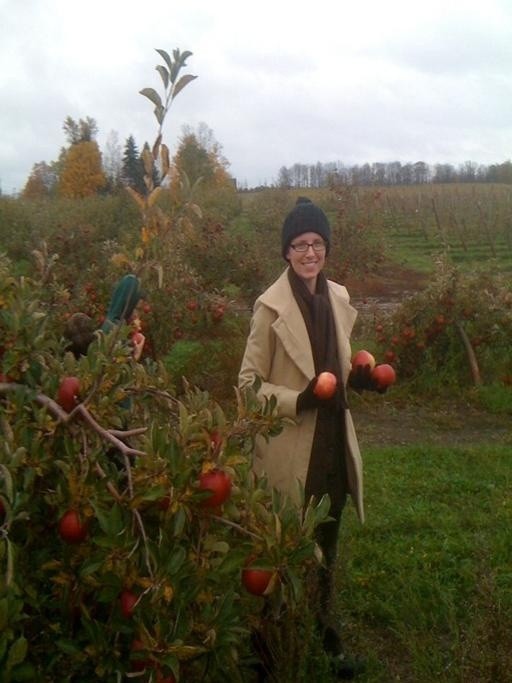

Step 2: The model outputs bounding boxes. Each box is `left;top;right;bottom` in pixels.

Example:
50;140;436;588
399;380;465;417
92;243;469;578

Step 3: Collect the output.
297;377;338;409
349;364;387;394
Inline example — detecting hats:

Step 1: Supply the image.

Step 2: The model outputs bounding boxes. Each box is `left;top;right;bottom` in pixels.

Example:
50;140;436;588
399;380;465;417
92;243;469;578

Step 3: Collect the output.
282;197;331;263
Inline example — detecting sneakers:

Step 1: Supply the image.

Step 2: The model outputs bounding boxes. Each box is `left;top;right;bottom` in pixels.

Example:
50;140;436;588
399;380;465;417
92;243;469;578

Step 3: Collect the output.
318;620;345;661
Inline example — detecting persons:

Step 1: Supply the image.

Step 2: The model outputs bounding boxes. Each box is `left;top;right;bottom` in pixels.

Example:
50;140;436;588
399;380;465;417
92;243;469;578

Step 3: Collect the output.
62;308;95;356
235;195;390;681
92;275;149;415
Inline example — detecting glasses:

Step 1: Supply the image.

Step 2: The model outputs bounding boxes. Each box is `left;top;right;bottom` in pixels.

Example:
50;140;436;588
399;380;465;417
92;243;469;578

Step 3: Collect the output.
290;240;328;252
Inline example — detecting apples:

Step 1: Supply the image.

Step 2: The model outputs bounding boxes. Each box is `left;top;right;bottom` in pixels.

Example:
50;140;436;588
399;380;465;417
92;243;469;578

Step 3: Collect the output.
351;349;376;373
313;372;336;402
0;284;280;683
372;256;512;388
